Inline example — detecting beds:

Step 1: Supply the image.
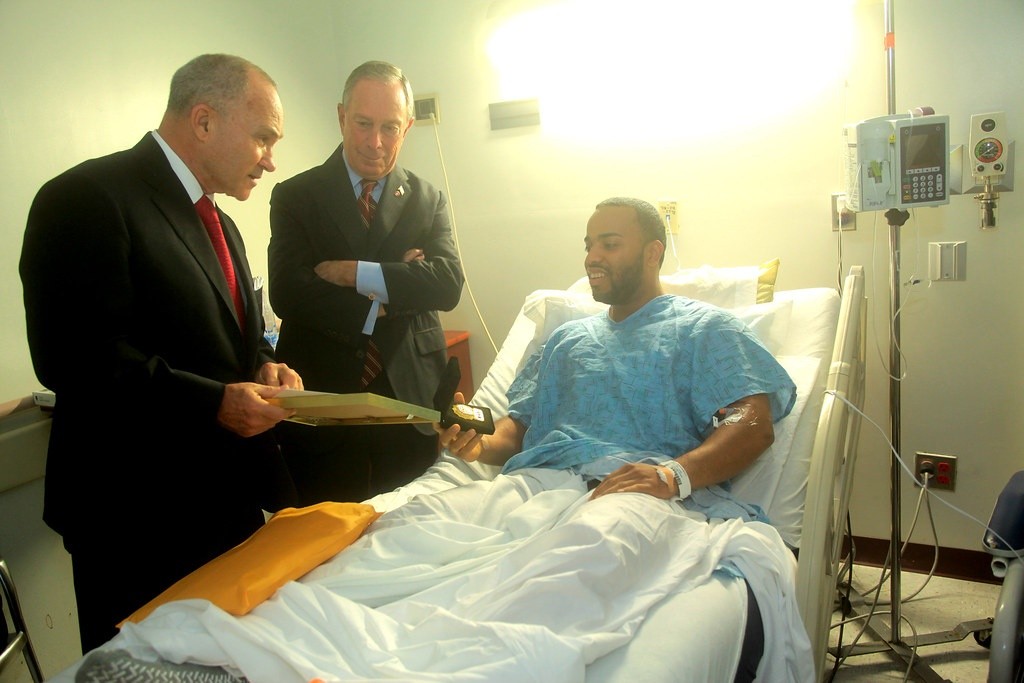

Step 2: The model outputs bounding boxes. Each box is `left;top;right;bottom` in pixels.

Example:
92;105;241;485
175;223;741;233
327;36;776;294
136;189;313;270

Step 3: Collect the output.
76;265;874;682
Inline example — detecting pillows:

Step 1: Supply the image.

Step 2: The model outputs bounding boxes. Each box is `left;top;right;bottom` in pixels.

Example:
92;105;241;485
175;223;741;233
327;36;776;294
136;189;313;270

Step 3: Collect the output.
520;288;776;360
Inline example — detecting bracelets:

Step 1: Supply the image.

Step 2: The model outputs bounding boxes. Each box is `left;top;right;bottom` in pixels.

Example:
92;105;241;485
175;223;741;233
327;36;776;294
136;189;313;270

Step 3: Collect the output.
660;460;691;499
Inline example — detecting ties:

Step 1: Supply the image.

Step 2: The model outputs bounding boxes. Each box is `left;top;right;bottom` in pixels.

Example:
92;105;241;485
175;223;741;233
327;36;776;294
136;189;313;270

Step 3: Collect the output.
357;178;383;390
194;194;244;334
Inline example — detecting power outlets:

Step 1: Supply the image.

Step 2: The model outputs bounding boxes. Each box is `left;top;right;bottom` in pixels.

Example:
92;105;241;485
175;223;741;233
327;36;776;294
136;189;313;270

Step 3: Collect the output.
915;451;956;492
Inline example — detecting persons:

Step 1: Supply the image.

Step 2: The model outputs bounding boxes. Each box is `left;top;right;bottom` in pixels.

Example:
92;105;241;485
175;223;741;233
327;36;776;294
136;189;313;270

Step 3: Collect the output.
268;60;464;506
74;196;797;683
18;53;303;658
